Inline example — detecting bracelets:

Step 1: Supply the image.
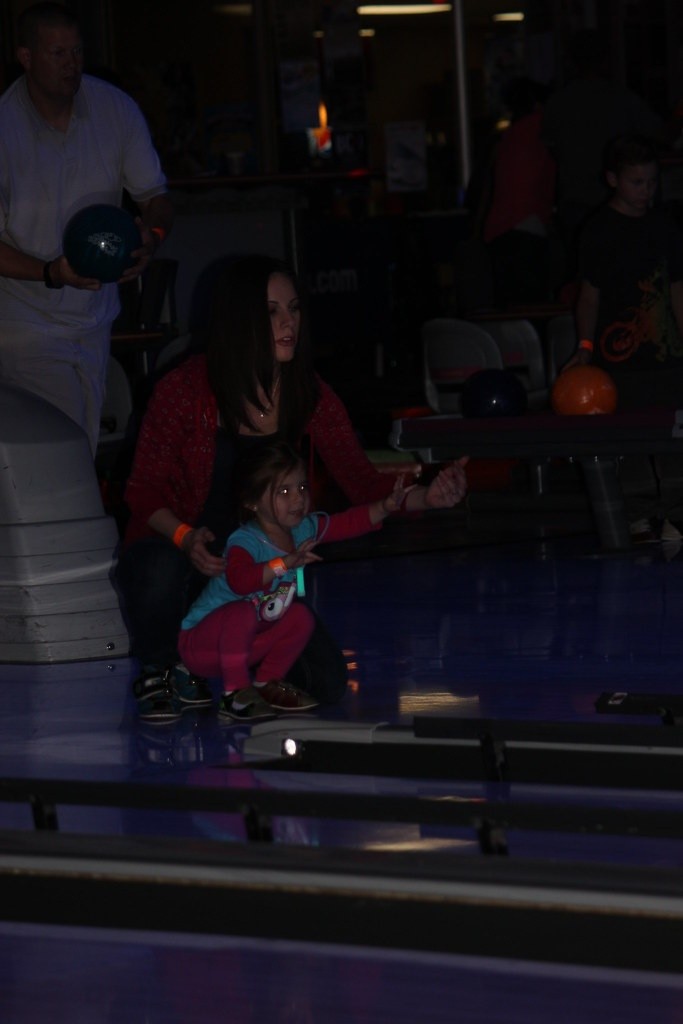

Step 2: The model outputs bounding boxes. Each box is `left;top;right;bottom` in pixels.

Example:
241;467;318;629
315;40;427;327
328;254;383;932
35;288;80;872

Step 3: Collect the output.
172;524;194;551
577;340;594;353
149;228;164;240
268;556;288;576
44;260;64;289
381;496;390;512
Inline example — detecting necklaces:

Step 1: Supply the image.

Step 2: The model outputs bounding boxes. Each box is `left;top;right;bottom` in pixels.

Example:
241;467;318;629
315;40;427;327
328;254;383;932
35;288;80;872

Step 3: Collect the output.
248;372;280;427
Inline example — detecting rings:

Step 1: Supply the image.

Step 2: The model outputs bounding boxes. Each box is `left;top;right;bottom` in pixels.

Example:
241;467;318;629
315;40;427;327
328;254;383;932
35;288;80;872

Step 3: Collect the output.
451;491;459;495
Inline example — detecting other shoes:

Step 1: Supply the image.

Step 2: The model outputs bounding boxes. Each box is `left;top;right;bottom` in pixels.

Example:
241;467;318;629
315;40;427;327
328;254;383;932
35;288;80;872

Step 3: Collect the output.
252;678;321;711
163;662;213;703
628;518;661;543
132;661;182;718
661;518;683;541
219;686;276;720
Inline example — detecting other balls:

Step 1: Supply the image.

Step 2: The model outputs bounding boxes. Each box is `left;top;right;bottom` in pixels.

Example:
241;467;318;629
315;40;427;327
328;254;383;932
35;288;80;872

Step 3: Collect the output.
62;204;143;283
458;369;529;420
550;366;618;417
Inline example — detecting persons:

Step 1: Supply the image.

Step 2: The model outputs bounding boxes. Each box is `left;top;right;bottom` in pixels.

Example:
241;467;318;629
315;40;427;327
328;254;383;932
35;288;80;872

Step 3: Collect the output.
540;42;663;235
109;255;470;718
0;9;172;499
562;135;683;373
177;436;417;721
484;76;553;238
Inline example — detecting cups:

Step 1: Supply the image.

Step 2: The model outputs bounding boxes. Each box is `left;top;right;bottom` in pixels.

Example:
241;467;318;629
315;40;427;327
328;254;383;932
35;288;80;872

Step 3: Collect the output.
226;152;246;177
